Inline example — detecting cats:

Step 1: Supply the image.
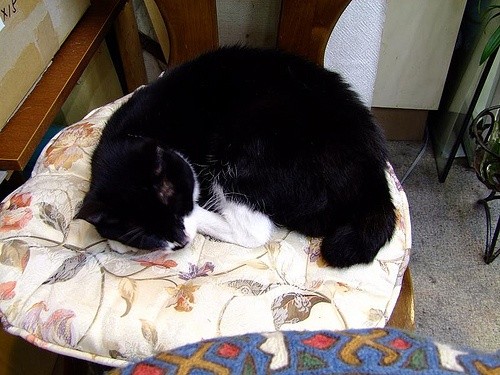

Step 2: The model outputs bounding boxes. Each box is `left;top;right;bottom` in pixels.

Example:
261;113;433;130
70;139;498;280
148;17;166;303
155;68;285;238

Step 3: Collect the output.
72;41;398;270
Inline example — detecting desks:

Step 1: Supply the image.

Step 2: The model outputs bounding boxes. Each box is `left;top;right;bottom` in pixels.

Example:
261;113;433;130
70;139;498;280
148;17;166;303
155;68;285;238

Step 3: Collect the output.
469;105;500;265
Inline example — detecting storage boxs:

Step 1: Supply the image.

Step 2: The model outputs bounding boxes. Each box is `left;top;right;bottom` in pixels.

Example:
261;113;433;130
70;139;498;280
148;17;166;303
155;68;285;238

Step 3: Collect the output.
0;0;91;133
54;38;124;126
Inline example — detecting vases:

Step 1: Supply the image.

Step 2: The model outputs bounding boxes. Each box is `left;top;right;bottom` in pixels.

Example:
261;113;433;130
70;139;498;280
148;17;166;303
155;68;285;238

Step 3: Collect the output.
480;108;500;184
